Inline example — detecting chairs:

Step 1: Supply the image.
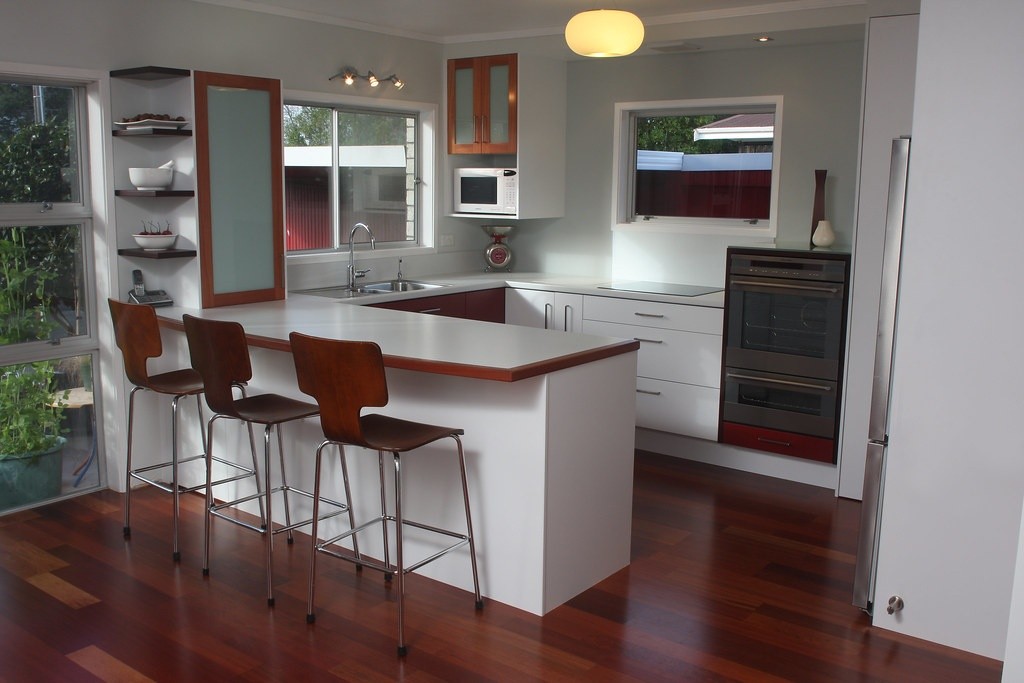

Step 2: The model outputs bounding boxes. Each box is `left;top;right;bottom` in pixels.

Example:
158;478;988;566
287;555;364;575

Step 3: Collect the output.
287;329;486;661
183;314;364;611
108;301;268;566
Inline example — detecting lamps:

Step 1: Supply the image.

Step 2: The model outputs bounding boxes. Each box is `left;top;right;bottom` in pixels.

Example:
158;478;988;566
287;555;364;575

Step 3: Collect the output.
564;0;645;58
327;66;405;90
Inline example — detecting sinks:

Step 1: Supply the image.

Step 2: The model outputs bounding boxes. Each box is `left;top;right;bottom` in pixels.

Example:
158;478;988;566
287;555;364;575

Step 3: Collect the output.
361;279;453;293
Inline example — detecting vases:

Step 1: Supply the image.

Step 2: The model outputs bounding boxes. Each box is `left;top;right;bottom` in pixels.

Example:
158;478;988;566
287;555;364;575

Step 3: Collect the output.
812;219;835;249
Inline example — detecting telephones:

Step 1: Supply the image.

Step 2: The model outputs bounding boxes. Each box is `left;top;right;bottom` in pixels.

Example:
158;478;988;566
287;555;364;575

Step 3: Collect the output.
128;270;174;308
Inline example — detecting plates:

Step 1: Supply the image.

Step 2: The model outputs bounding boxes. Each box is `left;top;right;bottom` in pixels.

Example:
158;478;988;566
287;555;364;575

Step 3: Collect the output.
114;119;189;129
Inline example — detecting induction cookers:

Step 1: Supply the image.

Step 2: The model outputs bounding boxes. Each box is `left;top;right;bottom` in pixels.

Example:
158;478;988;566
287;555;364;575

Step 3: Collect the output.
597;281;724;297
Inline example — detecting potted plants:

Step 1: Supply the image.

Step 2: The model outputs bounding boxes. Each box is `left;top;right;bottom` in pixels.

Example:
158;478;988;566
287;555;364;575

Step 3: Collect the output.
0;227;91;514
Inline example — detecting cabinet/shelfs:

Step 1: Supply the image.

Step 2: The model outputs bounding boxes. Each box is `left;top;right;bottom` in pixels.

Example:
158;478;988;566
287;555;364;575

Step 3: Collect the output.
364;293;466;320
447;53;518;156
504;287;583;334
582;295;725;442
109;66;198;260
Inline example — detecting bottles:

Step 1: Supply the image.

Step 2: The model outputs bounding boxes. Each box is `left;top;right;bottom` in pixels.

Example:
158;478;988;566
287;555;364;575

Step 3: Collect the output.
812;220;836;247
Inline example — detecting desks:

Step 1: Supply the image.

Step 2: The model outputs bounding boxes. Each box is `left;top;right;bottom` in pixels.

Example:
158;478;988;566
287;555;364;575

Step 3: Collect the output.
155;292;640;620
45;386;97;488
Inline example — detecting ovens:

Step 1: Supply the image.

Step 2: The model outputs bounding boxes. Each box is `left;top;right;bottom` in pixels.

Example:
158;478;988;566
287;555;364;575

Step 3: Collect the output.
718;245;852;465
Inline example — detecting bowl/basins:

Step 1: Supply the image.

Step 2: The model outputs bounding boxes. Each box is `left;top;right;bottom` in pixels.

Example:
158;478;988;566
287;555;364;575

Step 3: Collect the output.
129;168;173;190
132;233;178;251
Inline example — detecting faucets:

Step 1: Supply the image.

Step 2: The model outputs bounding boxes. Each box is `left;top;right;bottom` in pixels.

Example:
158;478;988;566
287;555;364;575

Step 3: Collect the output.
346;222;376;288
397;256;403;280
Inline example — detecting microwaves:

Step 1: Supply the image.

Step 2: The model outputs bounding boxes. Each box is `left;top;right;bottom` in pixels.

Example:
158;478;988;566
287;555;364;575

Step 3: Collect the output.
454;167;517;214
